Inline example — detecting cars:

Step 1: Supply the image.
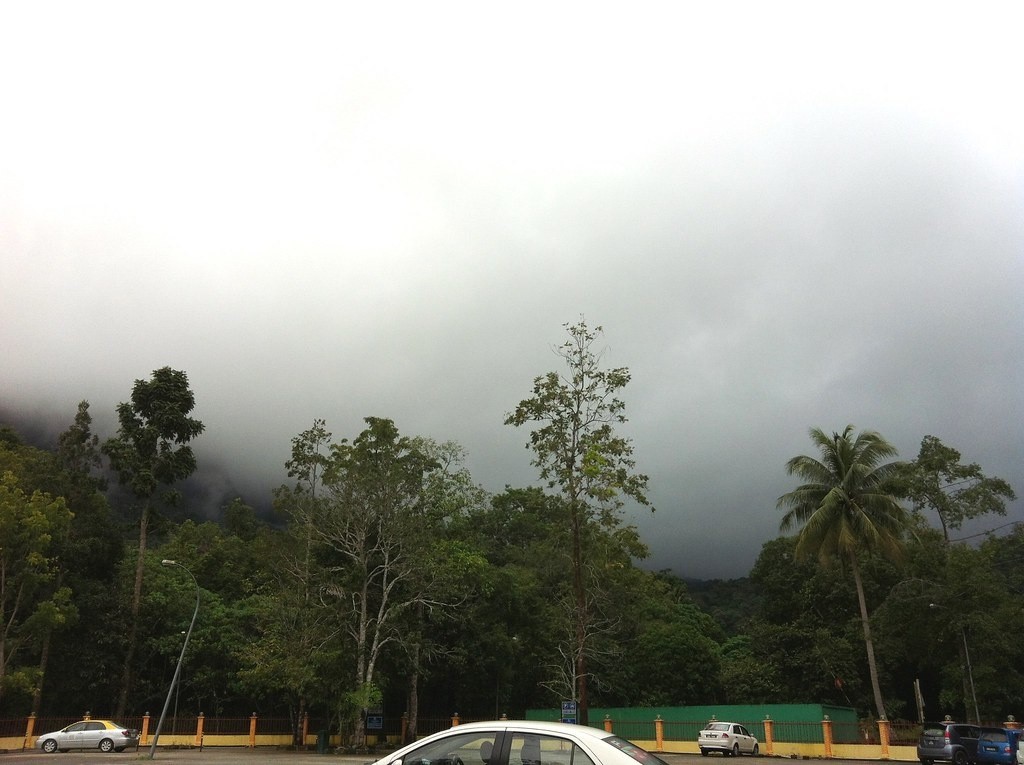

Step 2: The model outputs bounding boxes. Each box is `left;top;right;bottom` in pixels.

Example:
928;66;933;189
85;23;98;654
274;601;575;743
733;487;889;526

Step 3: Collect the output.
976;727;1022;765
1016;729;1024;765
698;722;759;757
35;720;141;753
370;720;669;765
917;722;984;765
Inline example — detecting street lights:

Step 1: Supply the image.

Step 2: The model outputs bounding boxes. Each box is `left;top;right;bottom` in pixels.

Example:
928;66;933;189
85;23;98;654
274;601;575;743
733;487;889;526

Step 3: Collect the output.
930;603;983;726
148;559;200;759
